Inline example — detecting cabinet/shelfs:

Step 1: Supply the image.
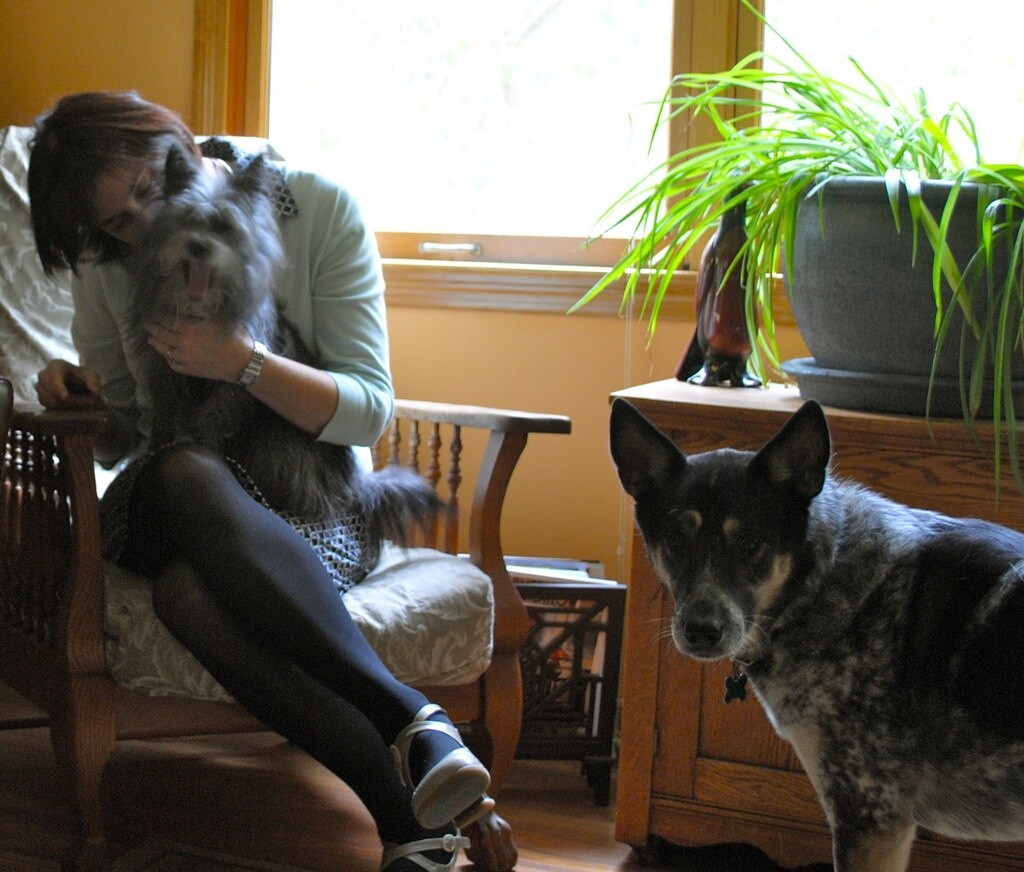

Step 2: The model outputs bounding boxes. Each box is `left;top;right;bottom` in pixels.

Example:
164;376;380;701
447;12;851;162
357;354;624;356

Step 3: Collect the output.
607;375;1024;872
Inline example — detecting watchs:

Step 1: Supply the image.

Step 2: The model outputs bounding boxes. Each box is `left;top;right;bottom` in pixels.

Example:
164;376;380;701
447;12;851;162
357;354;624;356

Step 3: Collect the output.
236;338;267;394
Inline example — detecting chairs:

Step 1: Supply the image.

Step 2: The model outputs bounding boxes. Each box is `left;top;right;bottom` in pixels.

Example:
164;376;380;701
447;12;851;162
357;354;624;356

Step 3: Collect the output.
0;125;571;872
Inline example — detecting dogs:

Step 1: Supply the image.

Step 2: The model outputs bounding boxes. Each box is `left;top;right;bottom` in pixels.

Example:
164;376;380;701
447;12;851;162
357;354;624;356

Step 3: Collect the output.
608;396;1024;871
47;145;453;546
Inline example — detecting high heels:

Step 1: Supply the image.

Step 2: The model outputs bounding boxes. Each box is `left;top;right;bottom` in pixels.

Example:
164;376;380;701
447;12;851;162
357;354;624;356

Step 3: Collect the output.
380;827;470;872
389;703;496;829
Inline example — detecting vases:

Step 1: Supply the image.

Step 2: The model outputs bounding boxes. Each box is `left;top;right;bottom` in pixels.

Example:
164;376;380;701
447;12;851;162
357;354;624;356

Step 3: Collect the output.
685;185;762;389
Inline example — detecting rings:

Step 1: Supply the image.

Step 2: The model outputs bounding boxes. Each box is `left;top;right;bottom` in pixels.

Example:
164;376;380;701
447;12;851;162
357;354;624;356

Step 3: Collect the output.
166;347;175;363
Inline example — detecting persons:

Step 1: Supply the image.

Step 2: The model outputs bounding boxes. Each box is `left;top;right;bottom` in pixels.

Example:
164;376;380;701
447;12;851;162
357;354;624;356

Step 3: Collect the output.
27;90;491;871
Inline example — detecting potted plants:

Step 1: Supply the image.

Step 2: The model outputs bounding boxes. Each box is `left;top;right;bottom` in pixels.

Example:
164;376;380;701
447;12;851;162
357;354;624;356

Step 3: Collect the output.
566;0;1024;512
